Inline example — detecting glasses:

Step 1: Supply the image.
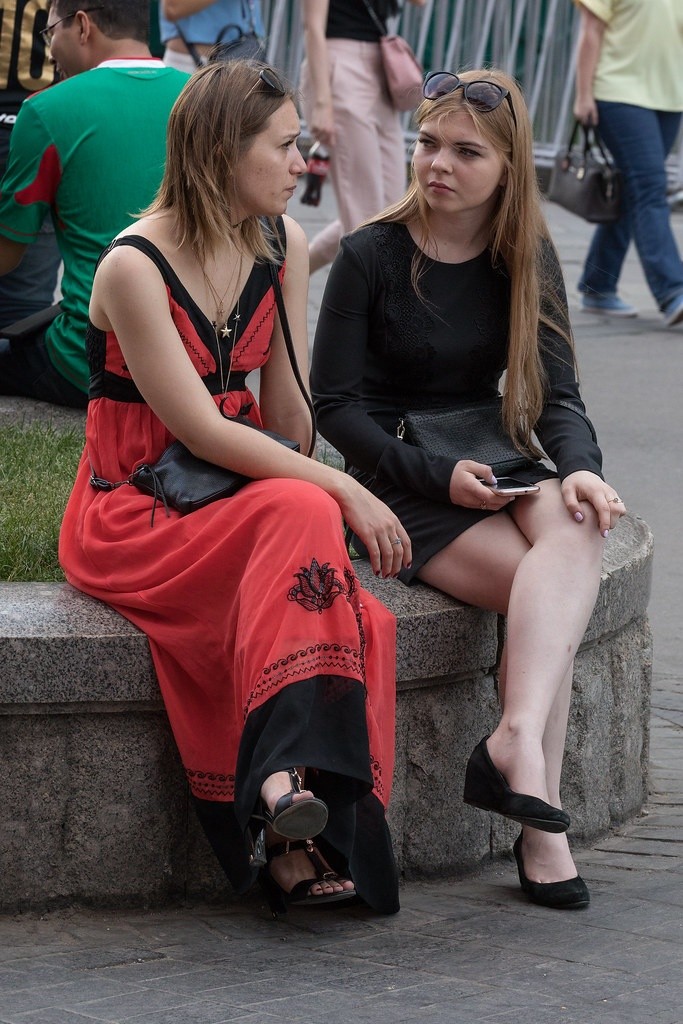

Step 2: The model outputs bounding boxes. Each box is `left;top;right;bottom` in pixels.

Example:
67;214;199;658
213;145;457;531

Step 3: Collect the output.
423;72;518;131
39;6;103;47
242;66;285;104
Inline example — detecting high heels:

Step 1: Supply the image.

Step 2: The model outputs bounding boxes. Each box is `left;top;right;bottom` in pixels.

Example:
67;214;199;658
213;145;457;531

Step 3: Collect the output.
245;767;328;867
251;839;355;921
464;735;571;833
513;831;591;909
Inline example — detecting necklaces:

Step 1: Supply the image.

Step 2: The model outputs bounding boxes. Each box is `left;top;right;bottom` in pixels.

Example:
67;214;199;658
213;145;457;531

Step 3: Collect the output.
183;221;244;402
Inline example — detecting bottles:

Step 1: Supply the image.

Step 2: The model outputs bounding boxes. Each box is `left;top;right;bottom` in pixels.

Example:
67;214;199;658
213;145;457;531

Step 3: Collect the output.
299;139;330;208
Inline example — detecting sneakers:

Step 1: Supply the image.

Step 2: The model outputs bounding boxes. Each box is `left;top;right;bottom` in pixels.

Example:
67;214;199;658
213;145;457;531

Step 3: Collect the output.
663;295;683;326
582;291;637;317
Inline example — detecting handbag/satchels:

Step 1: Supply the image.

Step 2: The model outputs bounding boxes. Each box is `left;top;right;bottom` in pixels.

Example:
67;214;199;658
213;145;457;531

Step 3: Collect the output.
131;417;301;528
381;34;427;112
209;25;263;66
400;398;542;477
547;121;622;222
203;24;266;65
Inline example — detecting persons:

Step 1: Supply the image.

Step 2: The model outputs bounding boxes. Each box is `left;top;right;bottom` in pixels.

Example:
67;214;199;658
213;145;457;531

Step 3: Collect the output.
56;57;414;918
299;0;427;276
0;0;196;412
574;0;683;325
311;69;629;910
159;0;266;64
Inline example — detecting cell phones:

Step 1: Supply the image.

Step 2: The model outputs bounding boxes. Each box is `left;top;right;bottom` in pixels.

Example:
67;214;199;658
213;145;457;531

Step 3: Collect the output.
477;477;540;497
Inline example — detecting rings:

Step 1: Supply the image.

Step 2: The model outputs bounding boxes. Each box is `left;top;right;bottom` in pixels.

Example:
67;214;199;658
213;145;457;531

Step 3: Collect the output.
481;501;487;510
621;499;624;502
392;539;402;546
609;497;618;503
